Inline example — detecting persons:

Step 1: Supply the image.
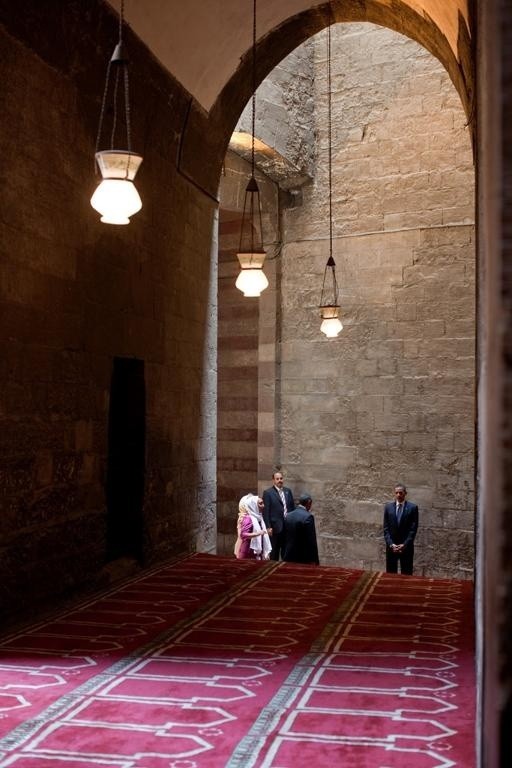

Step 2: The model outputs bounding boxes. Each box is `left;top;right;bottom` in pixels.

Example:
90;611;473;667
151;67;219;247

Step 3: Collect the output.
232;493;254;558
280;493;320;565
382;483;418;576
261;471;295;561
237;495;273;562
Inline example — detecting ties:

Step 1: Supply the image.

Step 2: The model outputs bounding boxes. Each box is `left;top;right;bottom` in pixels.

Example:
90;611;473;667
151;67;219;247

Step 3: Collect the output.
278;489;288;520
396;503;403;525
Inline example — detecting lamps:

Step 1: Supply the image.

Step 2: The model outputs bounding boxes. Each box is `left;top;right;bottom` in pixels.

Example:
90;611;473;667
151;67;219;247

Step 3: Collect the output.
90;0;144;226
318;3;344;337
233;1;268;302
